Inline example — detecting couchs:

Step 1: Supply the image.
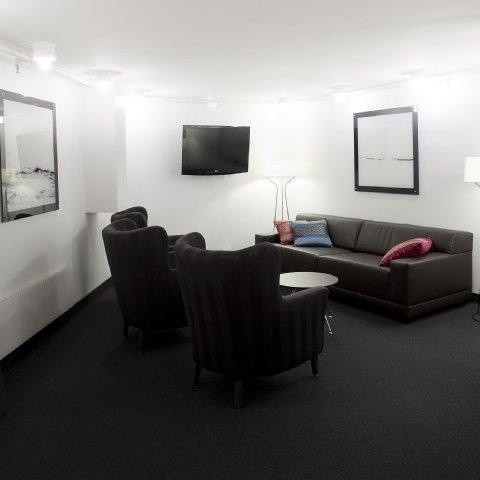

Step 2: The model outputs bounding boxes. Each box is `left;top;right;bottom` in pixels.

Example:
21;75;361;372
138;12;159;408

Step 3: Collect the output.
255;214;473;324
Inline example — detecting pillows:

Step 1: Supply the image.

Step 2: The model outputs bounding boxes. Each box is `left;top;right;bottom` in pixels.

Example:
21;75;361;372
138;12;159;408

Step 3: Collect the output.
273;219;333;247
379;236;433;266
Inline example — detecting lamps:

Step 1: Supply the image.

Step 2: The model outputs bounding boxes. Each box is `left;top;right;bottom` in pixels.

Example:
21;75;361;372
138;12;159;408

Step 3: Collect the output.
464;157;480;324
264;160;302;233
14;41;56;74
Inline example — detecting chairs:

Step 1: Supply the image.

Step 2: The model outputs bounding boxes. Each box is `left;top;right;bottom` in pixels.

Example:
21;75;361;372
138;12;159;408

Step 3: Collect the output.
102;218;189;352
175;232;330;408
111;207;184;249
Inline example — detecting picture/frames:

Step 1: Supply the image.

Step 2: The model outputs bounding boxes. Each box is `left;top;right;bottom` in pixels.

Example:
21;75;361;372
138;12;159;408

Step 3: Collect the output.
0;89;59;222
353;105;420;195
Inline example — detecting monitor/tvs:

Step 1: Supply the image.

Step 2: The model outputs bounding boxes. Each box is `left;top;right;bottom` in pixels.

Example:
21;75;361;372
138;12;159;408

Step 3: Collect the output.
182;125;250;175
